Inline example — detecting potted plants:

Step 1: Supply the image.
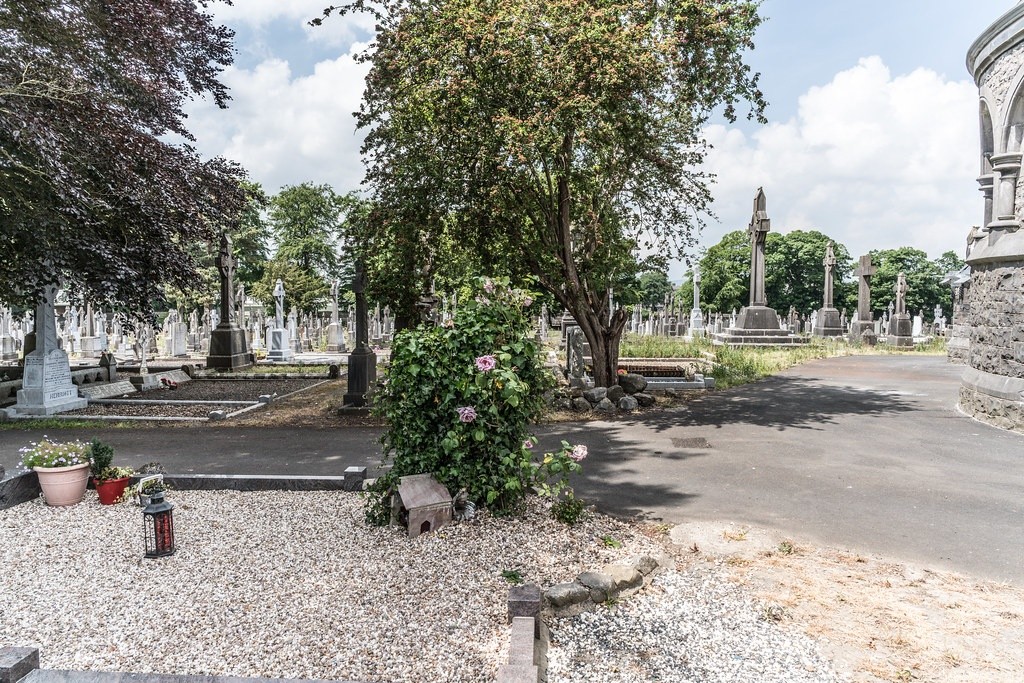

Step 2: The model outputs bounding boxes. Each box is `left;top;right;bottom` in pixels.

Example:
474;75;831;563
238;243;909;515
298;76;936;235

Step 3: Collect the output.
92;465;170;506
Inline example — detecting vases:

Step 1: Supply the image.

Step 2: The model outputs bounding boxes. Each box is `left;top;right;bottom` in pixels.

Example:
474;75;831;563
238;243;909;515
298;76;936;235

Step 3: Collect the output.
33;458;90;507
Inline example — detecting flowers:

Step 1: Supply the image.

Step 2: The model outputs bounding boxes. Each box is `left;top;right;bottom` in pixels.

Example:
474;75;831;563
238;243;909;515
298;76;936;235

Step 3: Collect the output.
15;435;93;475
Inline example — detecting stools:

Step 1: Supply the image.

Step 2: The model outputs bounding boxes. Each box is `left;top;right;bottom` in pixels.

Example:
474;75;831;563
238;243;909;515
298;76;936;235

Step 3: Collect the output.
389;473;452;539
